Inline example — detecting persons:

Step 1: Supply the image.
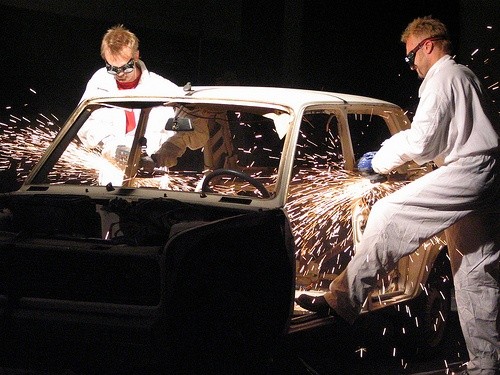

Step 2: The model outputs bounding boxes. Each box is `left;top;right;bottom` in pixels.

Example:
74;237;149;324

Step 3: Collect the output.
296;14;500;375
74;27;211;186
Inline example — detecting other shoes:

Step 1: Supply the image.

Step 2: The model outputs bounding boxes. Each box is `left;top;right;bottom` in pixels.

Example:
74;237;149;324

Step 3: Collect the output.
447;364;470;375
296;293;336;316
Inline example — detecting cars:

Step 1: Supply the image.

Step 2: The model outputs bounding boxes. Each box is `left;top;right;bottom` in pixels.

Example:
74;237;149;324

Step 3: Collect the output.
0;85;455;375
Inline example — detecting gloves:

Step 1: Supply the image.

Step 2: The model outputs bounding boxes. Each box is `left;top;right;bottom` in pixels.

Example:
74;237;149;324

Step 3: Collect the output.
357;152;376;171
158;141;179;167
116;145;129;166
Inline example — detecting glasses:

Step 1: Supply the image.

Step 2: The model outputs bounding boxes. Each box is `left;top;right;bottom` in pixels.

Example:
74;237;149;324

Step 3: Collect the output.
405;35;446;66
104;56;134;75
